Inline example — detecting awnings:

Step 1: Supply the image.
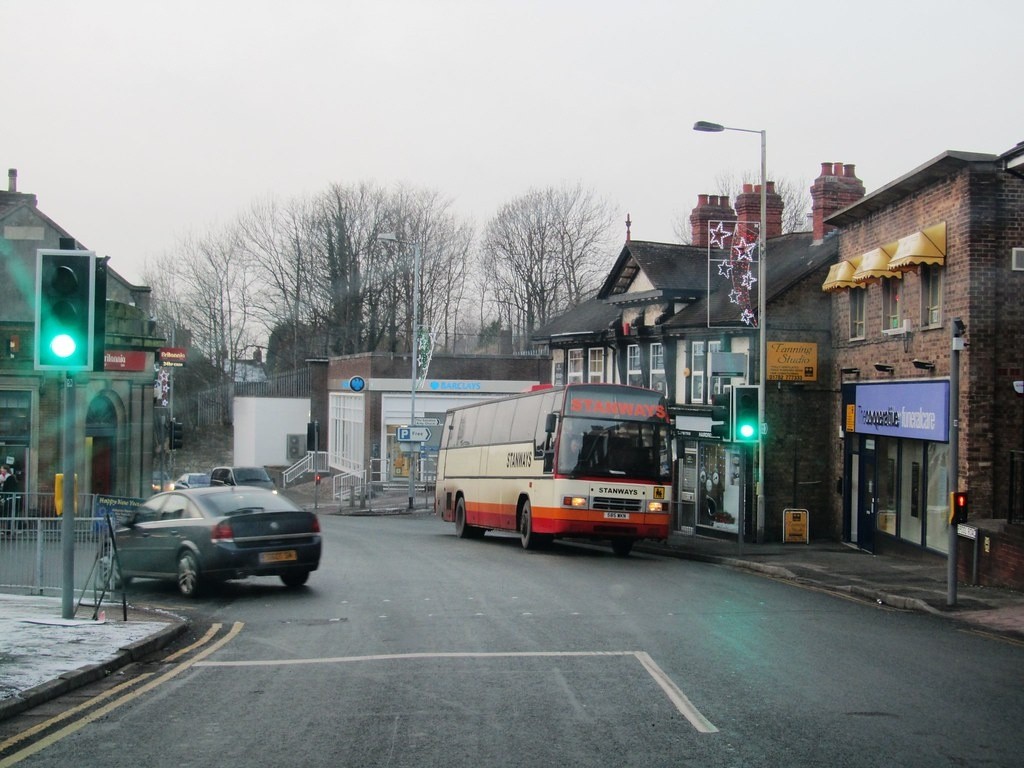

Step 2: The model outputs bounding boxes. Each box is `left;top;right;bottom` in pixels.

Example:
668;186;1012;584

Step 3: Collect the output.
820;220;947;295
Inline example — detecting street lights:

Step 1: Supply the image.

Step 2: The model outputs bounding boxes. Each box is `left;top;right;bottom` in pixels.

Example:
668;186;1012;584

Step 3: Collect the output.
692;119;767;543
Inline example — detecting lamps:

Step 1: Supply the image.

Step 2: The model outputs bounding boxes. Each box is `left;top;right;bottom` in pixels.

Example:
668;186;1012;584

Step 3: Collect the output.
912;358;935;369
873;363;894;373
841;367;860;374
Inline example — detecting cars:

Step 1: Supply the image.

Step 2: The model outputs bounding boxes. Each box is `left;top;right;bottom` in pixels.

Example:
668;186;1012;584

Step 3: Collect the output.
173;473;211;489
98;484;323;598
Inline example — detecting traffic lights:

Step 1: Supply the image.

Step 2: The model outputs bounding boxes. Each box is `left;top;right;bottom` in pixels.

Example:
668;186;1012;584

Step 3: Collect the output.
951;489;970;527
378;232;421;510
168;418;184;451
733;383;763;447
34;248;94;369
315;474;322;485
709;385;731;444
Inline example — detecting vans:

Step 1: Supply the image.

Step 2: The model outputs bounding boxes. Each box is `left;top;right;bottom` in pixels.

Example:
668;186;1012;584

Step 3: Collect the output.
208;465;278;492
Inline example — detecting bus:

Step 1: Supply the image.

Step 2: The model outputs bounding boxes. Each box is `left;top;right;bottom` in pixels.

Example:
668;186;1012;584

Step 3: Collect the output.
434;383;677;558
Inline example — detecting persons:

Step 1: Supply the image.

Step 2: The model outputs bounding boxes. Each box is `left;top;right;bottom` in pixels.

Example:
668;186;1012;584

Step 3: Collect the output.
561;439;584;471
